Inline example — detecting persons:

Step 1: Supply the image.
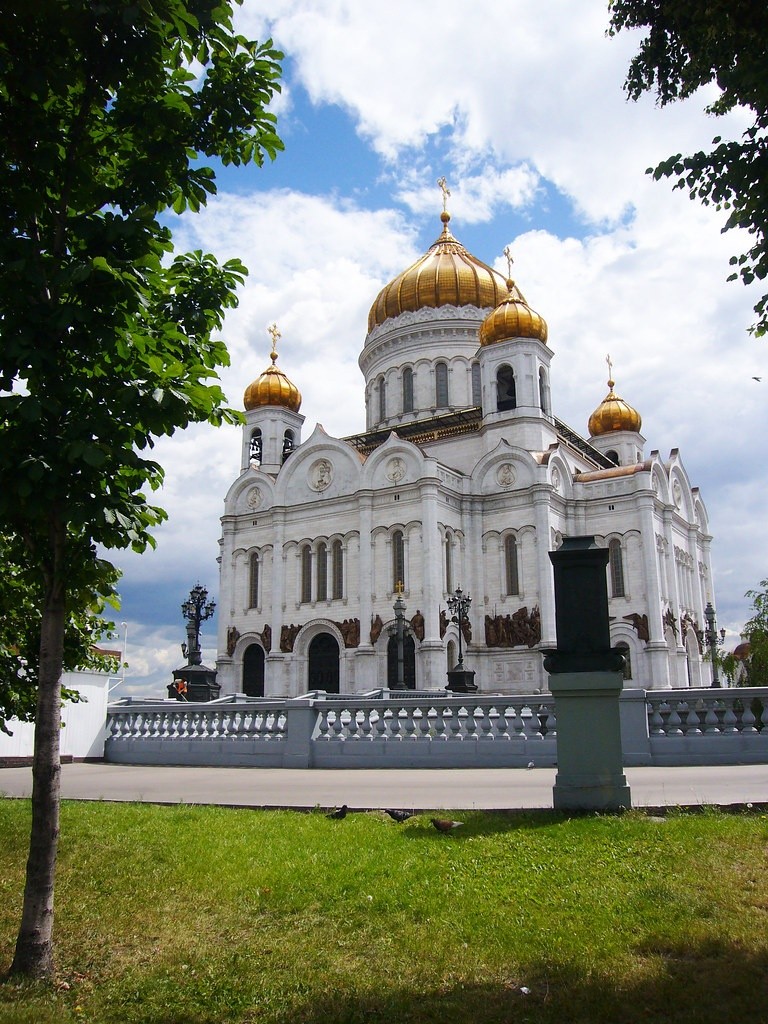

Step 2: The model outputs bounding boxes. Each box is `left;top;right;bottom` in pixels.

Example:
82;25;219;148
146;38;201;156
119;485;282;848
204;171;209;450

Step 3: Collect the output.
485;606;540;646
370;614;383;644
260;624;271;651
226;626;240;658
340;617;360;648
173;676;187;702
281;618;298;653
410;610;425;641
441;610;448;639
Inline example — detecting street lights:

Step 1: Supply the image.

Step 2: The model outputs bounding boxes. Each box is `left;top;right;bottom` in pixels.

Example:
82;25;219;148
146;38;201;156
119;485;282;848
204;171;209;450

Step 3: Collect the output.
444;583;480;694
390;581;412;690
171;578;223;702
121;621;129;683
704;601;727;687
